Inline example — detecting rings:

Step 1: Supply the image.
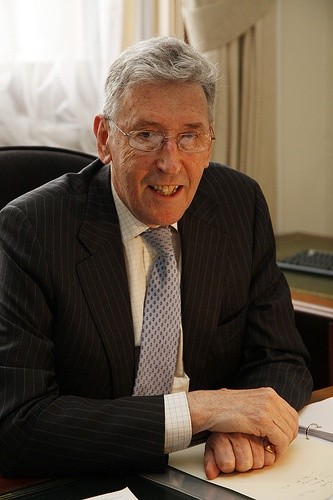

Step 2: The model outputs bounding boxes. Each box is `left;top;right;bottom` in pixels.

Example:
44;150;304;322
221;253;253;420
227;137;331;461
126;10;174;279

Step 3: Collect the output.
264;443;275;453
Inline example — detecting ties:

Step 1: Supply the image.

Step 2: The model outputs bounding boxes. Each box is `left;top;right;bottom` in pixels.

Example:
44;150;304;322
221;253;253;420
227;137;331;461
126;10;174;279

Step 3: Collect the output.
132;228;181;396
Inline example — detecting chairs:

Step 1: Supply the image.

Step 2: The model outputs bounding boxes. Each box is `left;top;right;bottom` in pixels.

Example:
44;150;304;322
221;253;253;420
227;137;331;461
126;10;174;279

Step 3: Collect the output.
0;146;103;210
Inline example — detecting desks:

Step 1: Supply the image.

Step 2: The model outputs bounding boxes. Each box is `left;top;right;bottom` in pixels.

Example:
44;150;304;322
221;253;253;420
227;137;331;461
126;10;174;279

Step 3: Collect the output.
0;231;333;500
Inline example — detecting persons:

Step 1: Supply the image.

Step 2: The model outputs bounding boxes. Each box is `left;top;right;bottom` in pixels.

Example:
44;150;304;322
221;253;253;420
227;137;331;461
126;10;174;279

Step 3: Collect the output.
0;36;314;500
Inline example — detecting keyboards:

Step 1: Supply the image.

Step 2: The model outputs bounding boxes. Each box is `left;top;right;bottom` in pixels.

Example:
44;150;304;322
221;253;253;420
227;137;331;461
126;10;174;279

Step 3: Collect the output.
277;248;333;275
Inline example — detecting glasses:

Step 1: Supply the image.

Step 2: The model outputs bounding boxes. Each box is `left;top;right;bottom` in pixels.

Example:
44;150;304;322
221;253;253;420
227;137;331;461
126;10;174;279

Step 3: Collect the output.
106;118;215;153
